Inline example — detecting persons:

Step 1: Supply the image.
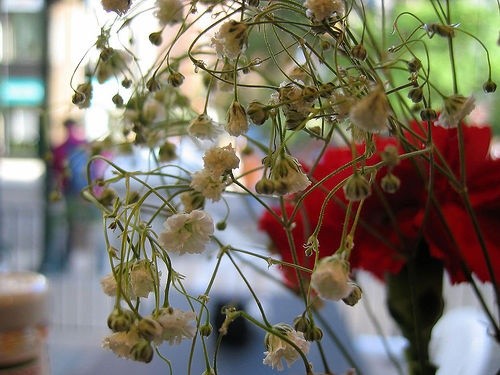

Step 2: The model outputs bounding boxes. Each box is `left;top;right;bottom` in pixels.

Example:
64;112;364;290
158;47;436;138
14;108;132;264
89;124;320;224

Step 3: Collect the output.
52;118;110;275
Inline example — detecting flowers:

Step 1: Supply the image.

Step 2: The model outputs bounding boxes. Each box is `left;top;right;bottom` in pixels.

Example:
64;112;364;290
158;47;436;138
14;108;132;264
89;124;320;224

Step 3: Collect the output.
261;114;500;375
